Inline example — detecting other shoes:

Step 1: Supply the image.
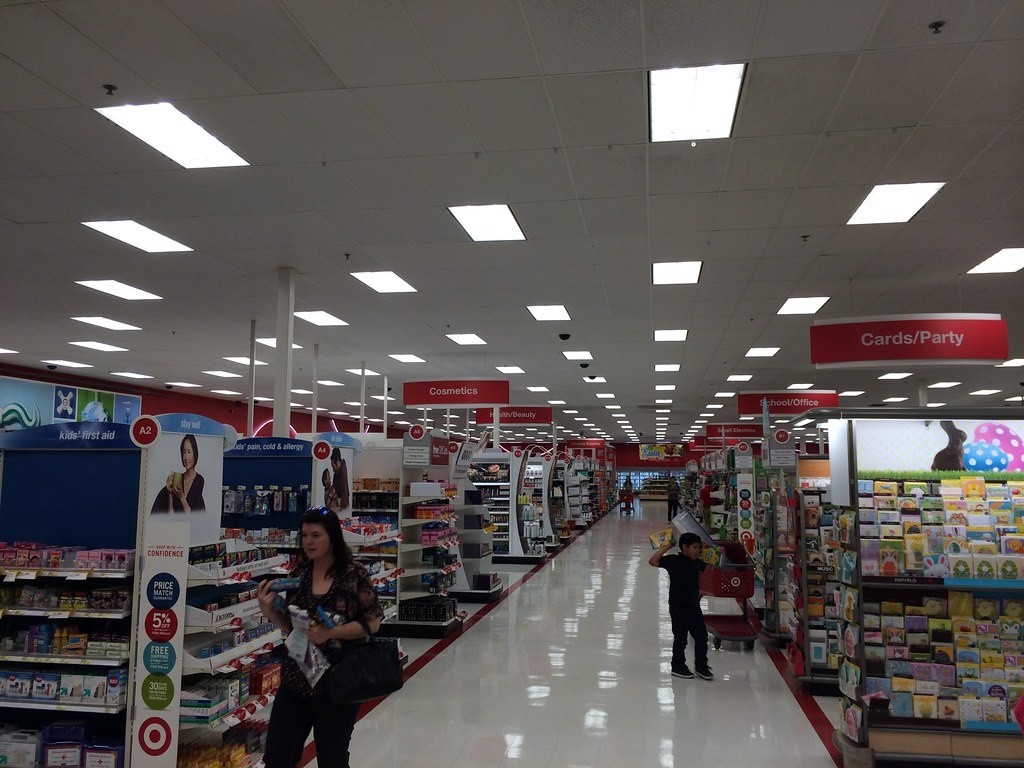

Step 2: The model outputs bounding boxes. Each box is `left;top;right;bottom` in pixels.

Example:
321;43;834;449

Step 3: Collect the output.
694;664;714;679
671;663;695;678
667;521;671;524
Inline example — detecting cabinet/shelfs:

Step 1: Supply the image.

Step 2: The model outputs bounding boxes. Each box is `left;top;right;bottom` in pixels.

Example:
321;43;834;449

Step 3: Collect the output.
793;487;843;697
448;504;504;604
338;527;400;639
351;490;400;512
465;461;616;565
0;566;136;714
853;470;1024;768
754;490;777;633
639;480;669;499
396;495;469;639
124;549;287;768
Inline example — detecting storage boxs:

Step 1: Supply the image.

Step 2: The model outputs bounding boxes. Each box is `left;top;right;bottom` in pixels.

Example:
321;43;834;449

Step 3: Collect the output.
1;478;498;768
802;476;1024;731
671;509;714;546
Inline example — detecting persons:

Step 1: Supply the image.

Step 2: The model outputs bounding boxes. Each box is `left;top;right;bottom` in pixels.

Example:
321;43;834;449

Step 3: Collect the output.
322;448;350;513
648;533;714;679
664;476;681;524
698;477;717;535
17;550;28;566
257;506;385;768
166;434;206;514
622;476;632;514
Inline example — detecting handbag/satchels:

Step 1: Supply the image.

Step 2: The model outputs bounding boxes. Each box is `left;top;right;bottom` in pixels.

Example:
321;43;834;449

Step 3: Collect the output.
331;619;404;702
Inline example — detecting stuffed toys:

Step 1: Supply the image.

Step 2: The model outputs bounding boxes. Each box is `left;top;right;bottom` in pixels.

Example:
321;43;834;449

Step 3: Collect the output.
924;555;949;578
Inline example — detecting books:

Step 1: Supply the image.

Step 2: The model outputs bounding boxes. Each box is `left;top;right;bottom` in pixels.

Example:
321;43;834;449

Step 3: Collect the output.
858;475;1024;729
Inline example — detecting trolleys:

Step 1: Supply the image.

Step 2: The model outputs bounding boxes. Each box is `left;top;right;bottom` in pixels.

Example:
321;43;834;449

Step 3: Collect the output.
619;487;635;513
699;541;762;651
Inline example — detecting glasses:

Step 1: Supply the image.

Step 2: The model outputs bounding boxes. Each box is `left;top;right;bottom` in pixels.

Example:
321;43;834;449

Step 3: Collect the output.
305;506;330;517
